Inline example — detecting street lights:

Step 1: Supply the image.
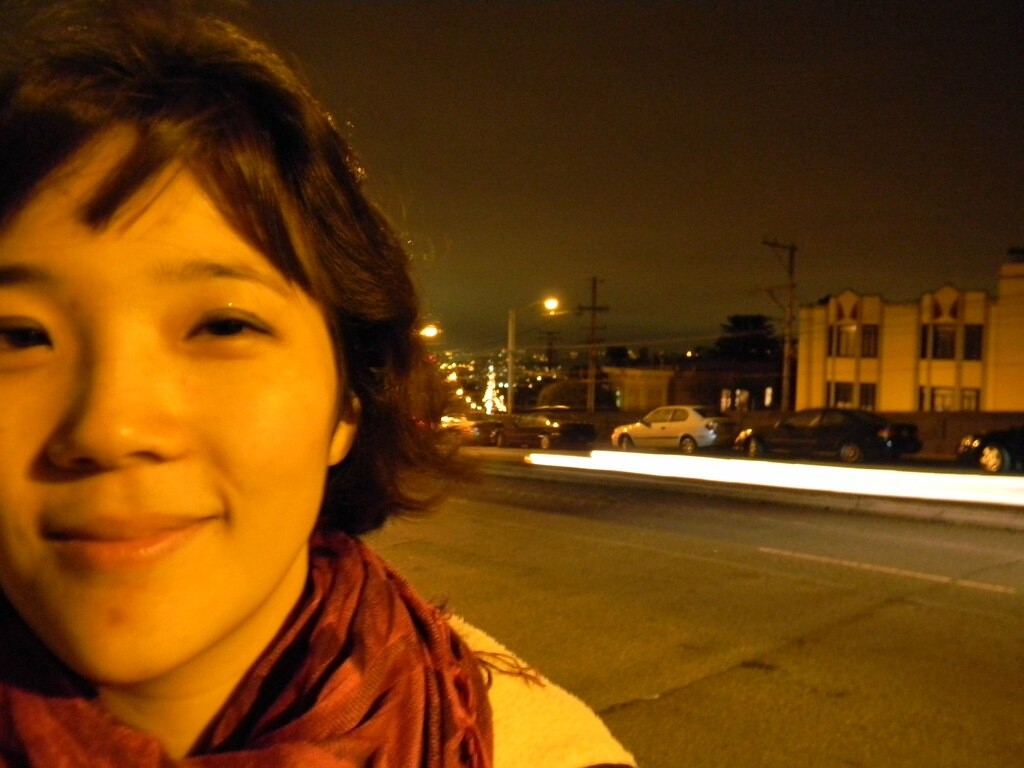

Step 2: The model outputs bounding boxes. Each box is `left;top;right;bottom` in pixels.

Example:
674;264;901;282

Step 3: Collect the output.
507;298;558;414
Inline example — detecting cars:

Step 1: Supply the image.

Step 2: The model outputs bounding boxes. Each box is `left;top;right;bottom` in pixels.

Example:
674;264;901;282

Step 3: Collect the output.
611;405;734;454
490;413;598;449
735;407;923;465
957;427;1024;473
441;414;505;445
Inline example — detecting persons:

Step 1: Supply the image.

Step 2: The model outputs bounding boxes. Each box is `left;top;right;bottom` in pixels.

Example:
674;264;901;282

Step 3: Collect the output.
0;0;637;768
